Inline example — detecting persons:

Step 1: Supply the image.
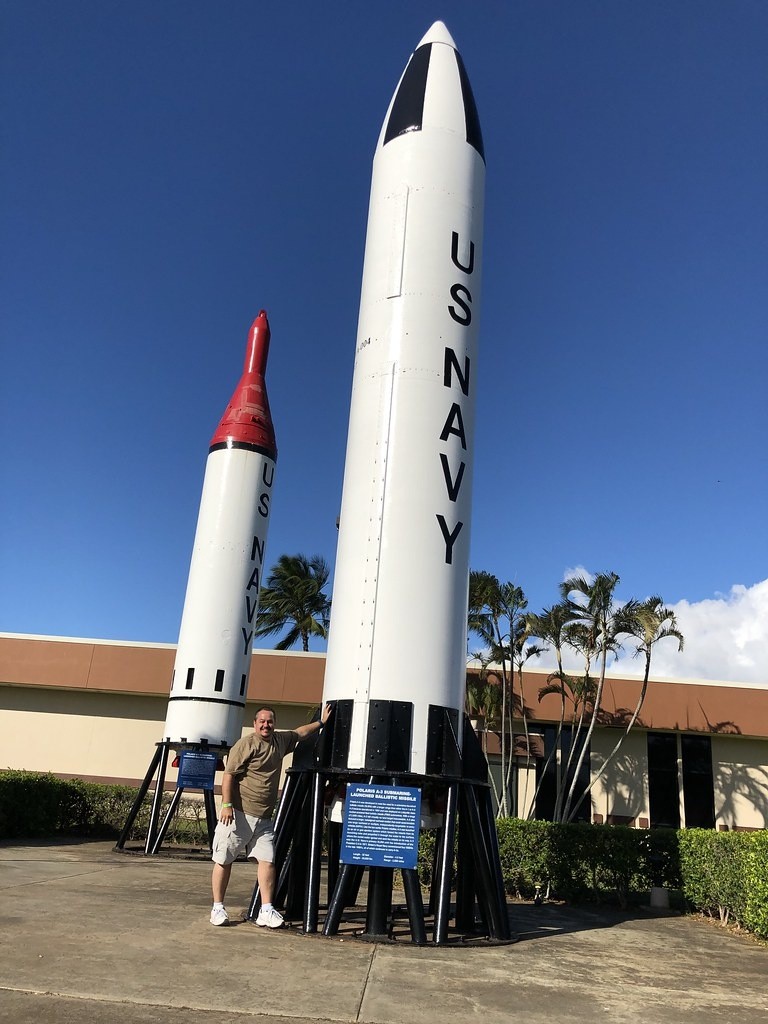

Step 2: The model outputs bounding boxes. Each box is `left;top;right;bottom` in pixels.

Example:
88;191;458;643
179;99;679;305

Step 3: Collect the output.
209;703;332;928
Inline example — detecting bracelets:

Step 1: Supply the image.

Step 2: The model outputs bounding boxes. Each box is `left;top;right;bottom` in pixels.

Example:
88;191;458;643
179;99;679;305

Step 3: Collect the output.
222;803;232;808
318;720;324;728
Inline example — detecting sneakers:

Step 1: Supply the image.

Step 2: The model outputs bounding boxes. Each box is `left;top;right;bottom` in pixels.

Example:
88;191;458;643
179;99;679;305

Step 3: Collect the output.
255;906;286;929
209;904;229;926
